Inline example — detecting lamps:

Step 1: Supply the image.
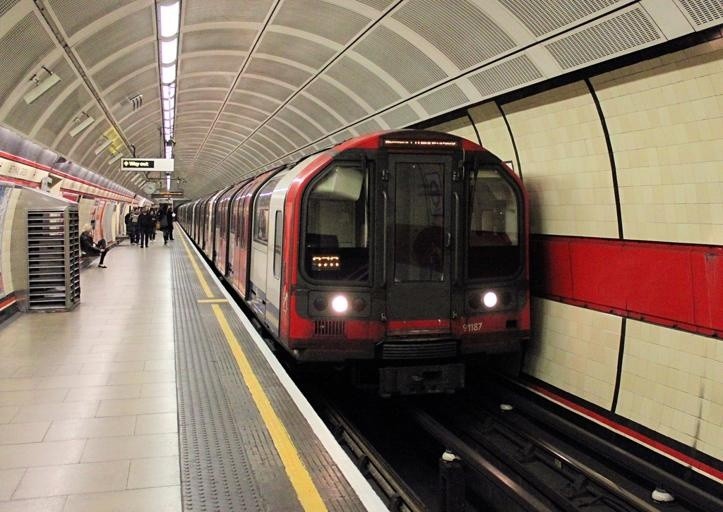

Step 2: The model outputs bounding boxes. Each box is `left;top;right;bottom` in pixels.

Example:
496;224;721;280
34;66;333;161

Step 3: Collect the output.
24;67;147;191
155;0;182;191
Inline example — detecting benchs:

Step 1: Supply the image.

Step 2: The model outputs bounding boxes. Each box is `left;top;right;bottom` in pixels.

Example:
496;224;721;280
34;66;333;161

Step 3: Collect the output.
79;238;120;267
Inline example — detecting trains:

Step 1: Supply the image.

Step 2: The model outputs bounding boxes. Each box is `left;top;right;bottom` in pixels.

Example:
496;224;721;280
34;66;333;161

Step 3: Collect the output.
177;126;536;401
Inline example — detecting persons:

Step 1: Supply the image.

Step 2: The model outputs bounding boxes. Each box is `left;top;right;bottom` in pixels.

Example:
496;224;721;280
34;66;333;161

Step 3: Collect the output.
124;201;175;247
79;223;110;269
